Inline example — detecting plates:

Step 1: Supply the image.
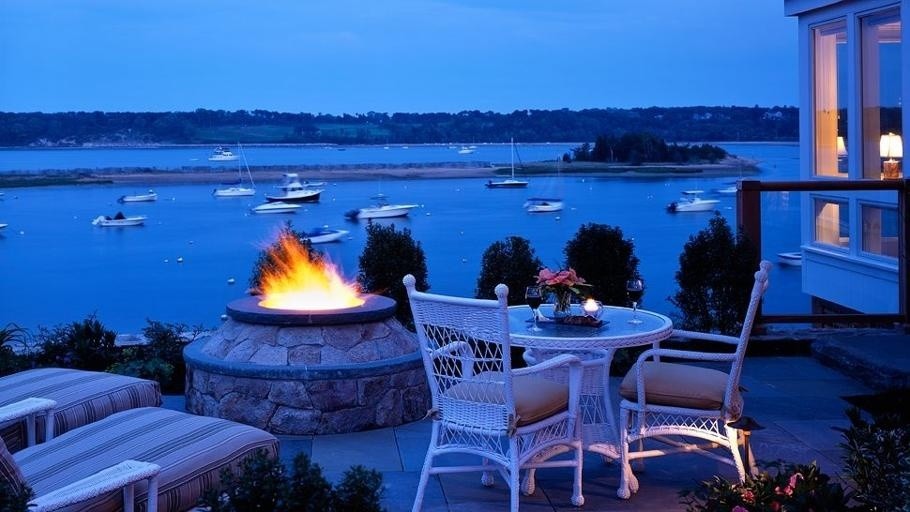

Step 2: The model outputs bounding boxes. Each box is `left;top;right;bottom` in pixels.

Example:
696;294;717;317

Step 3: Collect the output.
525;315;611;328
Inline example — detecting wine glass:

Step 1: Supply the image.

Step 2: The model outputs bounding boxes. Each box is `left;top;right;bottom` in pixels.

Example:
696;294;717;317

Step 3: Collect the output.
626;278;643;324
525;285;544;332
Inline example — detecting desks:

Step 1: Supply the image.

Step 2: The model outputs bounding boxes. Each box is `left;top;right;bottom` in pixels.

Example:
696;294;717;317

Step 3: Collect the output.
466;302;673;493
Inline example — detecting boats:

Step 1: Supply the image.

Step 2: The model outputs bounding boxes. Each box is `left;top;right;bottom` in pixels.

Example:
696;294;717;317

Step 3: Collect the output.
250;173;327;217
207;145;238;162
294;226;351;244
92;214;150;228
523;196;564;212
665;183;740;214
116;193;159;204
448;144;478;155
343;193;419;220
776;251;802;267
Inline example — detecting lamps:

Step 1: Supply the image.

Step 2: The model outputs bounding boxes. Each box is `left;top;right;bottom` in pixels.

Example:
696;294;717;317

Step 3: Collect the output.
879;133;903;179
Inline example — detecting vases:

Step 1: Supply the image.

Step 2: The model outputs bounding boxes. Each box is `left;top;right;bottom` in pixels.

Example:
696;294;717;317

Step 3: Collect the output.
553;290;572;318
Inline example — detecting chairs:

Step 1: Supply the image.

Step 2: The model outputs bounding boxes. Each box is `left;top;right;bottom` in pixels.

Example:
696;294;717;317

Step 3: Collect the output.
403;273;587;512
1;405;281;512
616;261;770;501
0;365;163;461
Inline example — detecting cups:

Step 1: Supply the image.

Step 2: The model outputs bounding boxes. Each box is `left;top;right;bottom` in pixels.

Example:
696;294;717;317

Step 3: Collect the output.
578;301;603;320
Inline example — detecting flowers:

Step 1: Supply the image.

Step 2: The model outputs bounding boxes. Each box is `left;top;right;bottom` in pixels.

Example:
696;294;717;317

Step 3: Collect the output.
535;263;595;295
676;460;862;512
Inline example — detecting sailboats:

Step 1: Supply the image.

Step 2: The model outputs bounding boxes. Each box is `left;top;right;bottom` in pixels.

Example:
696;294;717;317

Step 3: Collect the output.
210;142;258;198
483;136;529;188
